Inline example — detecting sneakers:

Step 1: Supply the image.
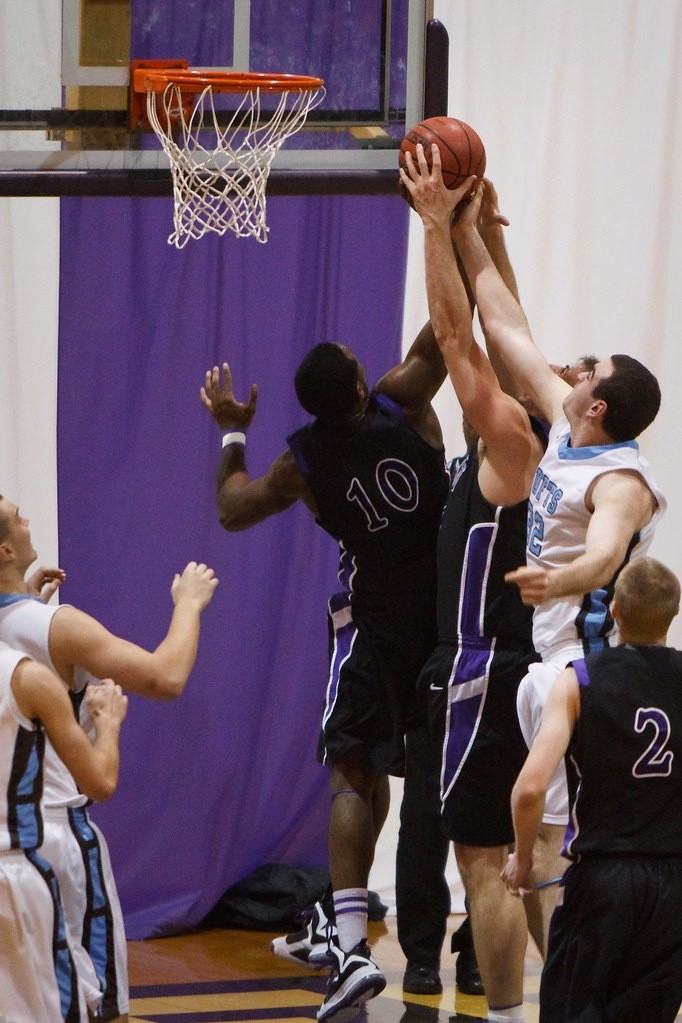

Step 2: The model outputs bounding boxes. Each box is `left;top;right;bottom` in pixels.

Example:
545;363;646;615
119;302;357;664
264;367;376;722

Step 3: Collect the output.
316;939;387;1023
269;901;339;971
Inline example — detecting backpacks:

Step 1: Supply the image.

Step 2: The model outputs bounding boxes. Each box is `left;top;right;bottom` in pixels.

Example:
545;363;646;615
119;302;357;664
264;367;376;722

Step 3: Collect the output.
208;864;388;934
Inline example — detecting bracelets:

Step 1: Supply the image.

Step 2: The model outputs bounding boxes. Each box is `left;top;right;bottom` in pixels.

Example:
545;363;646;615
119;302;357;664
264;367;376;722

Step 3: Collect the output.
222;432;247;448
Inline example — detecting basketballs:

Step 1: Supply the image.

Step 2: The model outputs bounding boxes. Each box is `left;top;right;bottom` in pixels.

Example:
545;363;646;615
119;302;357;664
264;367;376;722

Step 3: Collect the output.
397;114;488;203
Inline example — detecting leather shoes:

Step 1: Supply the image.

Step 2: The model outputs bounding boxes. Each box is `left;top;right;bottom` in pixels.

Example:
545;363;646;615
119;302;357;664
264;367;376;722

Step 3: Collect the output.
402;957;443;996
454;952;488;996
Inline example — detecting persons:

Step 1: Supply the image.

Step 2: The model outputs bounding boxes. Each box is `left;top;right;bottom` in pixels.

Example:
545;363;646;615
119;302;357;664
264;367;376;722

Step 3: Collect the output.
0;494;218;1023
199;143;669;1023
500;559;682;1023
